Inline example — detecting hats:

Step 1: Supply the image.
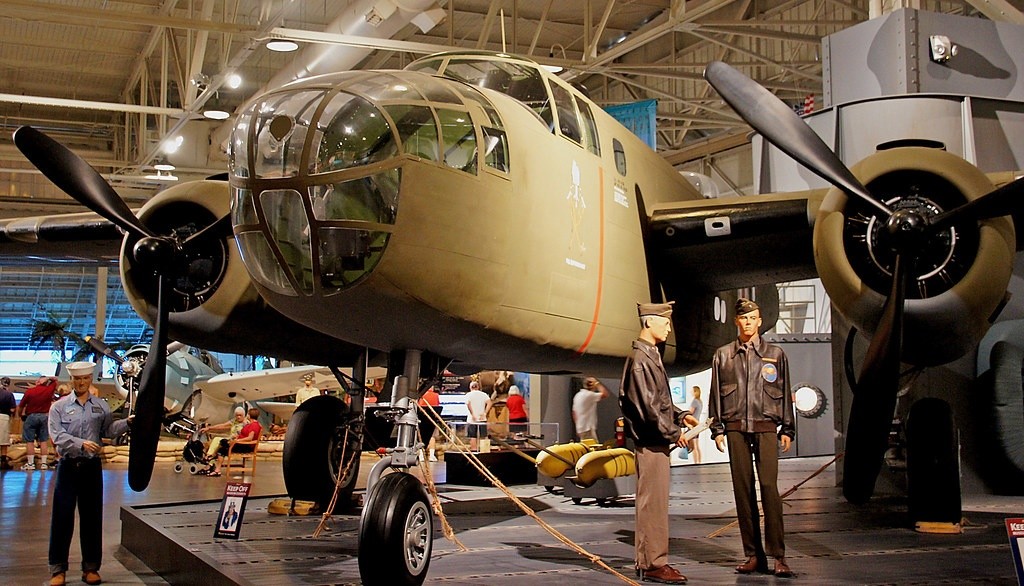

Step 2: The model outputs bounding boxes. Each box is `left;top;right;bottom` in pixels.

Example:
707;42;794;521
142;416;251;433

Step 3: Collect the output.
735;297;759;315
635;300;676;318
66;362;97;376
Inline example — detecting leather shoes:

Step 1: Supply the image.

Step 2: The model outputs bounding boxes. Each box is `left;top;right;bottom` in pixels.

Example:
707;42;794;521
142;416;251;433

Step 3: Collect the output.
643;564;688;583
773;559;791;576
734;554;769;573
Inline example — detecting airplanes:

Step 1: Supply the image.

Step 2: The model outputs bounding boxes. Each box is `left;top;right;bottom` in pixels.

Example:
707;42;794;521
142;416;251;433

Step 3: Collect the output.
0;28;1024;586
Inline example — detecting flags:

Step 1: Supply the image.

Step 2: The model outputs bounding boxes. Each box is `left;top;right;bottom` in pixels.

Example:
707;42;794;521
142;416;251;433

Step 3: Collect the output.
792;92;815;116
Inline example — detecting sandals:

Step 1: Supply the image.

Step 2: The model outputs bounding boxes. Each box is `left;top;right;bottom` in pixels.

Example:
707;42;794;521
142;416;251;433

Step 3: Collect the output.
197;468;213;474
207;471;222;477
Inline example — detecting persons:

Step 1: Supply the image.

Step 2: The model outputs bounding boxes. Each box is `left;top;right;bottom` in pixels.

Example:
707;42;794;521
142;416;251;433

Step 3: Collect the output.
465;382;493;439
687;385;703;464
616;300;702;584
47;384;73;469
707;299;796;578
505;385;529;448
0;376;17;472
418;383;441;462
295;379;320;407
18;375;59;469
45;362;136;585
571;377;609;445
197;406;262;475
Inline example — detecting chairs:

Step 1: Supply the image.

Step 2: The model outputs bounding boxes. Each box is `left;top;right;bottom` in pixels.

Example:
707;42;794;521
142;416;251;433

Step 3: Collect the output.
207;424;263;482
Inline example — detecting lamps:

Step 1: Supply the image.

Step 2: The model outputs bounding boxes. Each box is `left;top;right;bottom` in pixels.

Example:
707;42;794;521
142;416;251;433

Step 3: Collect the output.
265;0;299;52
539;44;570;73
153;154;175;171
201;90;231;119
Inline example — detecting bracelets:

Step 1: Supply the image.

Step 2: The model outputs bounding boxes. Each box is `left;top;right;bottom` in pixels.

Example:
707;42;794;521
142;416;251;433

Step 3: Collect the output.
594;381;601;386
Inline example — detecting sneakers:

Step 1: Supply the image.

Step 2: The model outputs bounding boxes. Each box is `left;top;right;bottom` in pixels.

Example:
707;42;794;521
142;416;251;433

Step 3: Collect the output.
81;571;101;584
20;463;36;470
41;463;48;470
51;571;66;586
